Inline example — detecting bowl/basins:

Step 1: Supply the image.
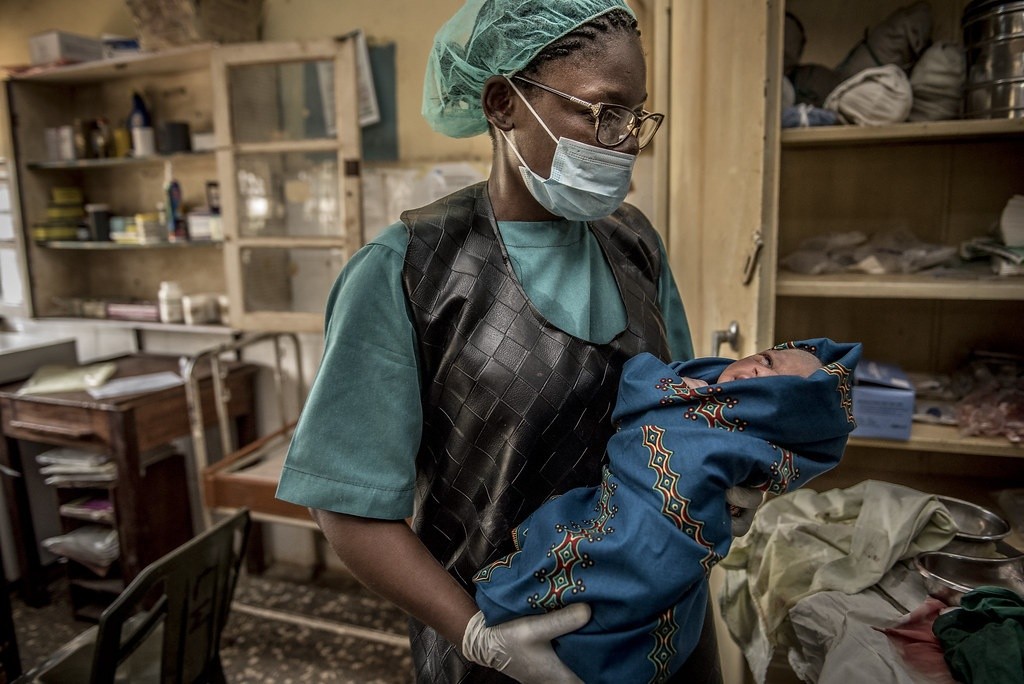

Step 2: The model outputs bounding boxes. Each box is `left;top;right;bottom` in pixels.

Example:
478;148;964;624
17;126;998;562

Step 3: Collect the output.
934;494;1011;557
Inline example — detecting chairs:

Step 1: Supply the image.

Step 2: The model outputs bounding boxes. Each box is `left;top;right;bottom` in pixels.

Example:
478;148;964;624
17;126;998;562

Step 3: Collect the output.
18;508;253;684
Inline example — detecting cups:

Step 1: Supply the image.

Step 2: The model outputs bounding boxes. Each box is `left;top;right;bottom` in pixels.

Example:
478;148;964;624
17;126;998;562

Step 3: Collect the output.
88;205;110;241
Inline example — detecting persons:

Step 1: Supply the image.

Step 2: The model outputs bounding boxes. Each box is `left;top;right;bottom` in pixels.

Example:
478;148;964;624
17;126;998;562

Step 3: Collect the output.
474;338;862;684
274;0;724;684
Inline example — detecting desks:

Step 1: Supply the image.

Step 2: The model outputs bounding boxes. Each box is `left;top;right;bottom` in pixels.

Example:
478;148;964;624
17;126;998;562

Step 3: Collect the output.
0;351;265;624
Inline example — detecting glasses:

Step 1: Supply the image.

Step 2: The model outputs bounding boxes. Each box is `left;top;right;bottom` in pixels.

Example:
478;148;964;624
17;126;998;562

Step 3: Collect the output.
507;76;665;147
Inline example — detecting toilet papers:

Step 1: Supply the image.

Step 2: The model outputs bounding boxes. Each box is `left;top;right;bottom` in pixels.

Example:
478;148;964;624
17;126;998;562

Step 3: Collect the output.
131;128;155;158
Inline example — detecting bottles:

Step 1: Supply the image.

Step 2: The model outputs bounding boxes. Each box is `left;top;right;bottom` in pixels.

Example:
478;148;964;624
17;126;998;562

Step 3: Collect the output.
164;161;185;243
127;94;152;153
158;281;182;324
98;118;114;158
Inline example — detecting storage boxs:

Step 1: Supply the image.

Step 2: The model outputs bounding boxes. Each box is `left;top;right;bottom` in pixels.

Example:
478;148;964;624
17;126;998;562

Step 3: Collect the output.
848;355;913;440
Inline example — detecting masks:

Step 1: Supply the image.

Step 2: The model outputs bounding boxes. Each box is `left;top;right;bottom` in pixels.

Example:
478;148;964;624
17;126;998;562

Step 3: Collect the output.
485;75;636;220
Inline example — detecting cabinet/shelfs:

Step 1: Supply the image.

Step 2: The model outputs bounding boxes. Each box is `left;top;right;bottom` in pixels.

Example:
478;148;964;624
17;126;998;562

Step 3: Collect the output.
652;0;1024;554
0;27;366;537
